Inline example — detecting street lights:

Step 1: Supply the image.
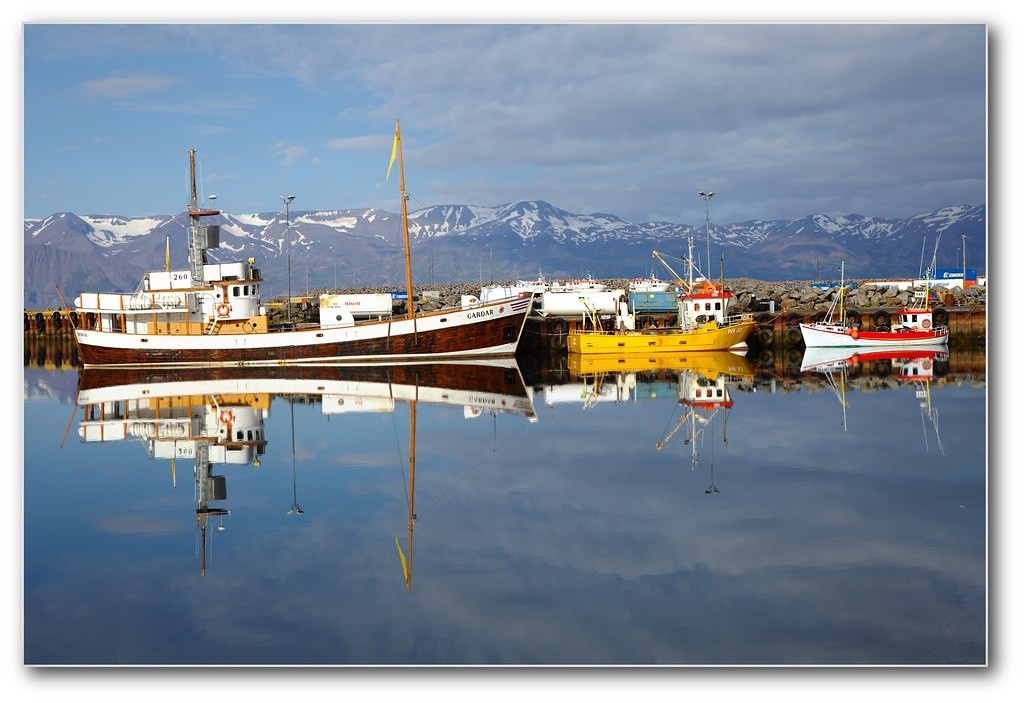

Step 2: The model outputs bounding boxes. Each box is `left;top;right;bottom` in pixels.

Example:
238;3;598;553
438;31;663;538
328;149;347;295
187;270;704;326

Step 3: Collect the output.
698;192;716;282
703;412;721;494
287;394;304;515
278;195;295;322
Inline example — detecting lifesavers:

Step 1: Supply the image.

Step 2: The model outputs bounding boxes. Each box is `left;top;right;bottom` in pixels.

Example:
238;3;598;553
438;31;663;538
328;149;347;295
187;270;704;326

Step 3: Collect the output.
641;316;655;329
933;360;950;377
787;312;802;342
24;311;95;331
551;318;567;349
757;314;775;344
220;412;233;424
844;309;861;329
217;302;230;317
873;309;889;332
922;318;931;328
923;361;932;369
551;349;894;383
815;311;833;323
932;308;949;326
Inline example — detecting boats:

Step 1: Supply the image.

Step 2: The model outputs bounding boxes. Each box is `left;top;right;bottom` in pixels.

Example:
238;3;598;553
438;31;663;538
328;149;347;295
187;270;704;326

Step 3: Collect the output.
568;352;757;381
653;244;733;325
799;261;949;348
655;370;734;469
800;344;949;432
567;316;756;353
461;277;626;316
628;274;670;292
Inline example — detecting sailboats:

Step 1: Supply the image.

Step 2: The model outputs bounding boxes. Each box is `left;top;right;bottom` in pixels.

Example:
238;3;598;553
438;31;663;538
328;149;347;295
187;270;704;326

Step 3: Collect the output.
60;358;539;594
55;118;535;369
